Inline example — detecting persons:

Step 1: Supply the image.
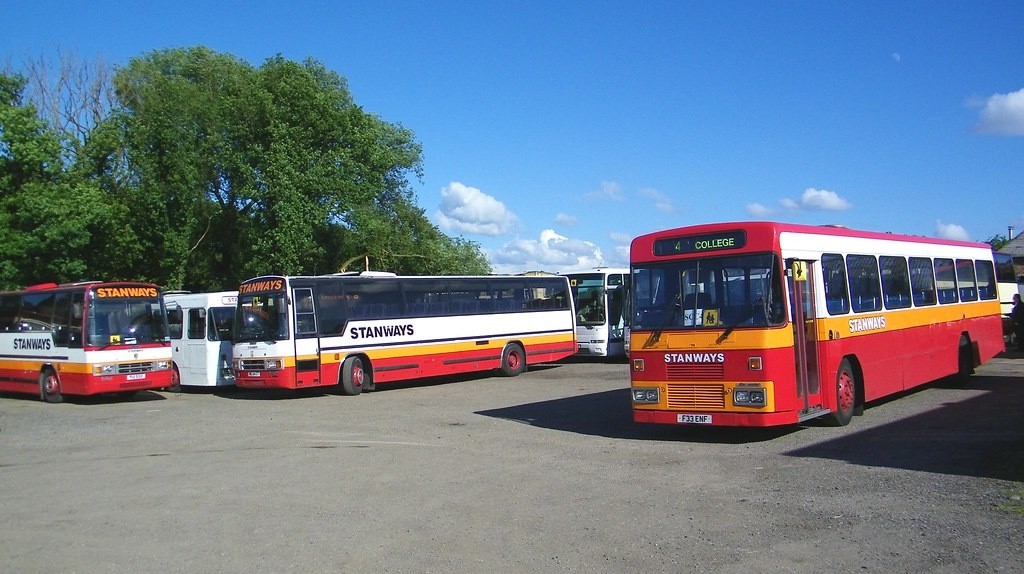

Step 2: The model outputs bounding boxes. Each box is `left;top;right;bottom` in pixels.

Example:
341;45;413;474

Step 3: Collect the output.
584;304;599;323
1002;293;1024;352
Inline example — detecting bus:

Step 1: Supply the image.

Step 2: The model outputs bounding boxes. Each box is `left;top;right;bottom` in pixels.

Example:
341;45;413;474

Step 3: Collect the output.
1;281;173;400
162;289;276;392
626;220;1007;427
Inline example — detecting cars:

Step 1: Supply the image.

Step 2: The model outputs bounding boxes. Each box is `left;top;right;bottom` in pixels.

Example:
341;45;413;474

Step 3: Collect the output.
993;251;1019;322
561;268;645;358
232;269;579;396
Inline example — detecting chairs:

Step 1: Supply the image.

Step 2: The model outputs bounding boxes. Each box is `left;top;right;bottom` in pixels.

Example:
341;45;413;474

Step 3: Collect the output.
296;295;560;335
109;309;128;337
785;285;989;321
